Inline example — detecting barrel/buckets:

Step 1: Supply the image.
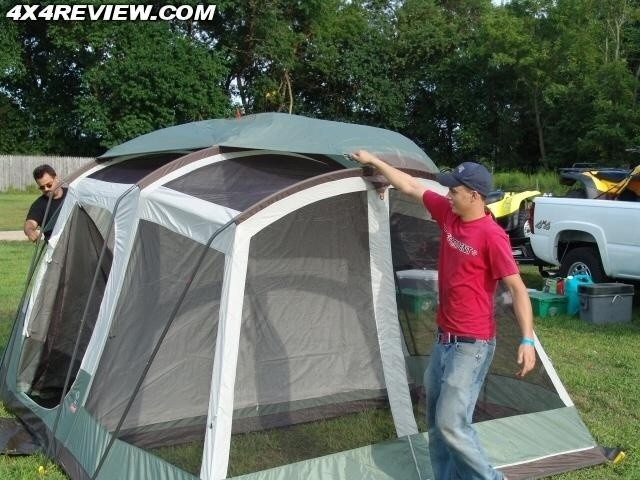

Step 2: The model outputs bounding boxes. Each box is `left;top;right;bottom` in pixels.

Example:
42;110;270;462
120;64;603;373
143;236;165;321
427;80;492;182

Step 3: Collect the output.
564;275;592;317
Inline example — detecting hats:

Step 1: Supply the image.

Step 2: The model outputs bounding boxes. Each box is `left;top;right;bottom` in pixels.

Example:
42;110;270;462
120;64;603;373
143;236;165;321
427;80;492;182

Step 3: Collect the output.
436;162;492;197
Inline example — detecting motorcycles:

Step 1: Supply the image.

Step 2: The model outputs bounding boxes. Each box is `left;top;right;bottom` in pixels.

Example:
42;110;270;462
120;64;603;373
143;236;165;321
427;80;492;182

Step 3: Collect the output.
486;185;540;236
559;147;640;200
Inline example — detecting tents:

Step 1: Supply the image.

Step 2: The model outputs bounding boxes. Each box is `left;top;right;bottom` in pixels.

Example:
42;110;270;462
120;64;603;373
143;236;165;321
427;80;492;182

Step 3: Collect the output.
1;110;628;480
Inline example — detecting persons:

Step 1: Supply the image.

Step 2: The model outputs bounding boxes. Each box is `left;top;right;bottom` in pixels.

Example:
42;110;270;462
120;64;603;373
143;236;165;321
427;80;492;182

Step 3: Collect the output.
23;158;70;245
342;147;538;480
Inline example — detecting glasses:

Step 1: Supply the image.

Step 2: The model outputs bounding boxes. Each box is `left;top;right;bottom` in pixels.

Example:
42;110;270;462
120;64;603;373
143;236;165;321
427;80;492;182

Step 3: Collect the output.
40;179;54;190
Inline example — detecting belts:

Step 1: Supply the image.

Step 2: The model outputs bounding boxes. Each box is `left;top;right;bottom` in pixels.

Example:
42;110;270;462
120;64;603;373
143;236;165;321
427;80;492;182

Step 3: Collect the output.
436;332;488;343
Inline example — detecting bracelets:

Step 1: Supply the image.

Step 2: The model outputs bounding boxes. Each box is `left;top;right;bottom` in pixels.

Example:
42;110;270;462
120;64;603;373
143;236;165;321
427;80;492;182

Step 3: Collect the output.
520;338;536;346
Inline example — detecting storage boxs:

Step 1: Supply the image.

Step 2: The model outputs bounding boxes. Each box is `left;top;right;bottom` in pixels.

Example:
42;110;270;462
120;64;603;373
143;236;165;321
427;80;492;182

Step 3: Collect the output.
526;280;636;321
395;269;439;315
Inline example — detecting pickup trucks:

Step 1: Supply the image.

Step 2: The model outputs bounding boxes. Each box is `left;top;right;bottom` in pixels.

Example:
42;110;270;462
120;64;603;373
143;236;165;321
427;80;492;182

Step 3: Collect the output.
524;197;640;284
